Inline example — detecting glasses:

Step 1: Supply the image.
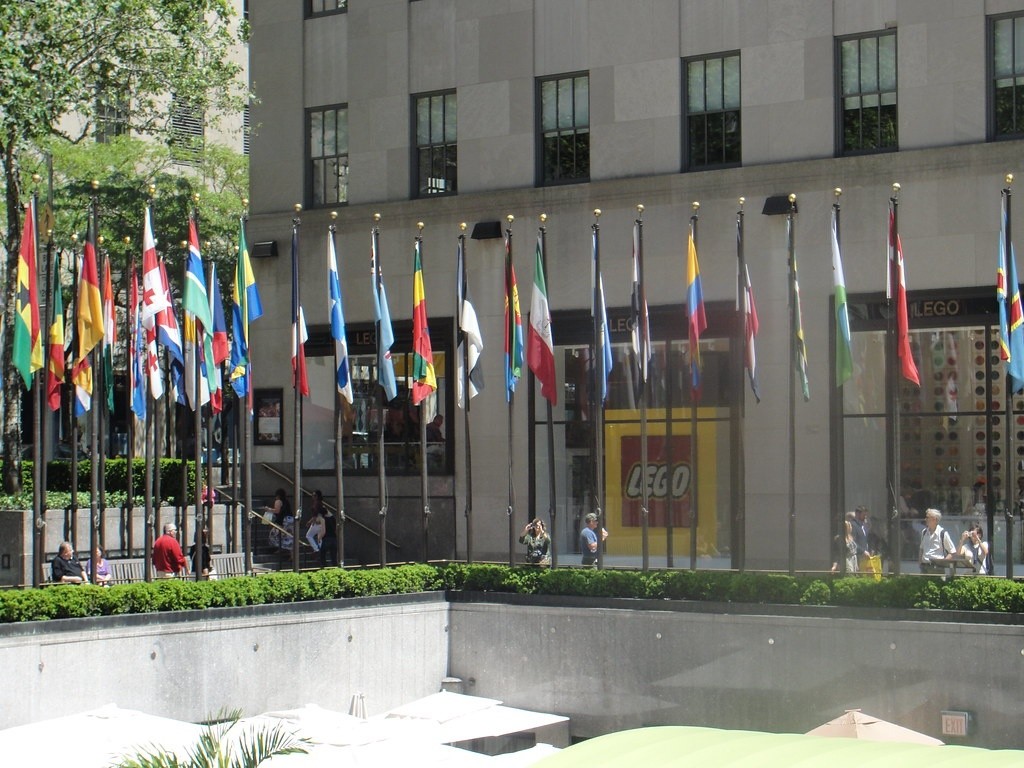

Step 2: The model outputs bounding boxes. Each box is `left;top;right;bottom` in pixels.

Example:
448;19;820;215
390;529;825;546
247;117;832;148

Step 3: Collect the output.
312;494;316;497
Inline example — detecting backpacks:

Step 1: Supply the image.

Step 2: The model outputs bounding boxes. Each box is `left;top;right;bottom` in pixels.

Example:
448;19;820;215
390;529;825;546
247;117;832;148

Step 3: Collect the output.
976;543;994;576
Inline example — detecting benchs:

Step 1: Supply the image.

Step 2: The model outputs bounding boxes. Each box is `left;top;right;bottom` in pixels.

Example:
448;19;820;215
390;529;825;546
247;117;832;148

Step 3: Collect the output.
41;551;253;588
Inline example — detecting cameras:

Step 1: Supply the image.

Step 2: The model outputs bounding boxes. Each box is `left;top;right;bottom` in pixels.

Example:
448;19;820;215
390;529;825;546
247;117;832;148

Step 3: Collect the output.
968;532;974;537
531;523;537;528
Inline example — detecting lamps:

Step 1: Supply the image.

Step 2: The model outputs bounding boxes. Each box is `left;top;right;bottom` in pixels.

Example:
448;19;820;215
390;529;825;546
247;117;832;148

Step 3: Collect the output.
761;196;798;216
250;240;278;258
470;221;503;239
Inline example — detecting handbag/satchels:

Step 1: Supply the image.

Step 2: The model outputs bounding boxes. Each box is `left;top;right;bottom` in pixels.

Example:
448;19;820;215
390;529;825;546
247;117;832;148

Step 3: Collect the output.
261;511;273;526
858;556;881;582
531;550;543;562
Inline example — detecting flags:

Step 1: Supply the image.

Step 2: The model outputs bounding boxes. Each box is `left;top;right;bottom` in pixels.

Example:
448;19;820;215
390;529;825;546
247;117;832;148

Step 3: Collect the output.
291;224;398;404
734;210;921;405
44;205;117;418
128;207;263;423
997;196;1024;393
412;237;614;406
10;199;44;390
630;221;652;385
685;222;708;402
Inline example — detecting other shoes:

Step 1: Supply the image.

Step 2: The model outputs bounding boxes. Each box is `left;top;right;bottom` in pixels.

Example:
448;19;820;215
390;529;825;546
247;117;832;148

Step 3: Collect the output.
272;549;279;554
287;557;295;562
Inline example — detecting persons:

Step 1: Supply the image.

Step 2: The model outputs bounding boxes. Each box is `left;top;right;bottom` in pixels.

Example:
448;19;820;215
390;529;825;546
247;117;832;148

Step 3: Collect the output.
957;525;994;575
52;541;88;584
580;513;608;565
153;522;190;580
832;504;873;571
519;518;552;564
86;545;112;587
306;490;326;553
426;415;446;442
919;508;956;574
190;531;213;581
320;507;338;566
262;488;295;561
